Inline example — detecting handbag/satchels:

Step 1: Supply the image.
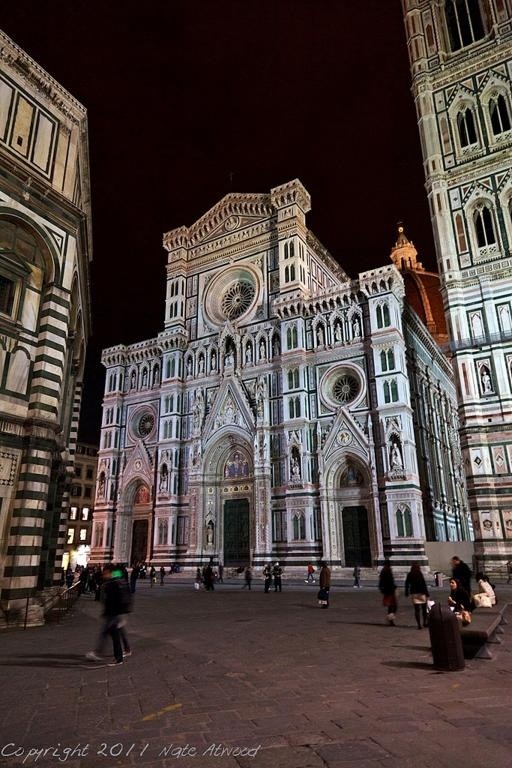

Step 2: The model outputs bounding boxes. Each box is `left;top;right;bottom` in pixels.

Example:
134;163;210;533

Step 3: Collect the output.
473;592;492;608
382;595;393;607
317;589;328;605
460;610;471;626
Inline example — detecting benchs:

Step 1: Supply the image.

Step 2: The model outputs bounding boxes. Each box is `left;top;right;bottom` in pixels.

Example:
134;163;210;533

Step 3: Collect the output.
459;599;510;660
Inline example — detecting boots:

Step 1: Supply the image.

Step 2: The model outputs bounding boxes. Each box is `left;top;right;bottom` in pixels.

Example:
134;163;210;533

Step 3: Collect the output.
386;613;397;626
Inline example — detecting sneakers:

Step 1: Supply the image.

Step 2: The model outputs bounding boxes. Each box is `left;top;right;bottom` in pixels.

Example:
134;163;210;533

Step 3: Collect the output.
122;651;131;657
353;585;363;589
417;623;428;630
86;652;103;660
107;659;124;666
304;580;315;584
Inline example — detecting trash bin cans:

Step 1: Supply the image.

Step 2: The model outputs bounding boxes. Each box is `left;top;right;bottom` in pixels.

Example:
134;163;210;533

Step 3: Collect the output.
434;572;443;588
428;601;466;671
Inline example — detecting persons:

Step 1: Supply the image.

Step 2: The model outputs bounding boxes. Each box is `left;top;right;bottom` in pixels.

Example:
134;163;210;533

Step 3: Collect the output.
317;561;331;609
353;563;363;588
304;561;316;584
380;557;494;630
64;559;178;668
241;567;252;590
193;563;224;592
264;562;283;592
